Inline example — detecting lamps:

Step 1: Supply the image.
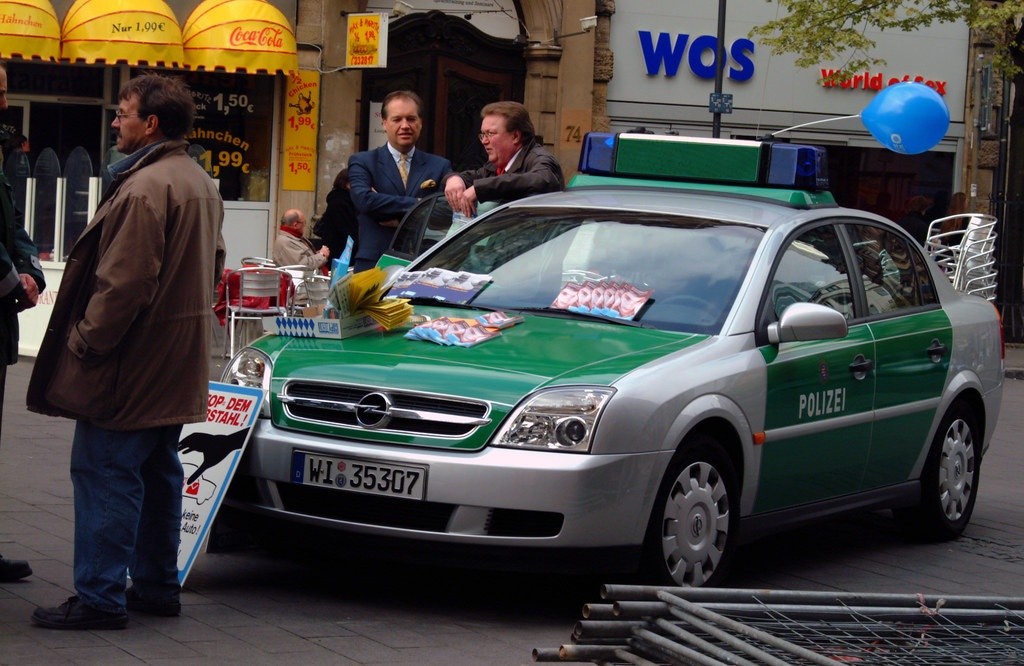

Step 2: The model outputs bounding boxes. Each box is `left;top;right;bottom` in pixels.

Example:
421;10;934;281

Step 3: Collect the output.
513;34;542;47
551;15;598;45
339;0;415;19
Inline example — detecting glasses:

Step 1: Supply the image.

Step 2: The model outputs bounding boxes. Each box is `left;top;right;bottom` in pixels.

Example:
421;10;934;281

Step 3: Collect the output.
479;133;512;141
116;111;140;122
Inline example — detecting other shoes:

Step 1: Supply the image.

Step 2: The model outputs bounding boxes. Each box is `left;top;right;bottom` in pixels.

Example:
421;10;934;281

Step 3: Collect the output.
0;555;33;581
126;587;181;616
31;595;129;630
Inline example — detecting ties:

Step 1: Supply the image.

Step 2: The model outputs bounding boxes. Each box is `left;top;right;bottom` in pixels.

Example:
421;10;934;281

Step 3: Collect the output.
399;153;408;187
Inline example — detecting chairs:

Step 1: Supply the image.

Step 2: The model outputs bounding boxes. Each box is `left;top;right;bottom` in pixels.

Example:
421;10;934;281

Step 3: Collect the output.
289;274;332;319
219;267;298;359
924;212;999;304
241;257;280;269
275;263;311;317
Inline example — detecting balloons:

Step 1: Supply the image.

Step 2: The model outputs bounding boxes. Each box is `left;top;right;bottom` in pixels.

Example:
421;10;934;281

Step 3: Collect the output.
860;82;950;154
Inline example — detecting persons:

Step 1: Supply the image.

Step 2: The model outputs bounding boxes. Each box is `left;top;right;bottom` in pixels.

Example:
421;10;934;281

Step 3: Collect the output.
24;74;225;631
442;100;565;220
853;174;973;268
6;132;30;222
271;207;329;275
0;59;45;581
310;168;359;259
348;90;452;274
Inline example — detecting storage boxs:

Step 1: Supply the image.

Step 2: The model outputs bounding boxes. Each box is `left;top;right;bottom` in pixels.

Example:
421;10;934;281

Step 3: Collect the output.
262;312;383;339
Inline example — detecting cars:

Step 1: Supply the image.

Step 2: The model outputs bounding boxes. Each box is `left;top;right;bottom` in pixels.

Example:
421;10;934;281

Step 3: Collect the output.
219;125;1005;595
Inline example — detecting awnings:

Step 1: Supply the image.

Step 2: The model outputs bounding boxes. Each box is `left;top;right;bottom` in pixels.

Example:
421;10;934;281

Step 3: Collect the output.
62;0;185;69
182;0;299;74
0;0;61;62
